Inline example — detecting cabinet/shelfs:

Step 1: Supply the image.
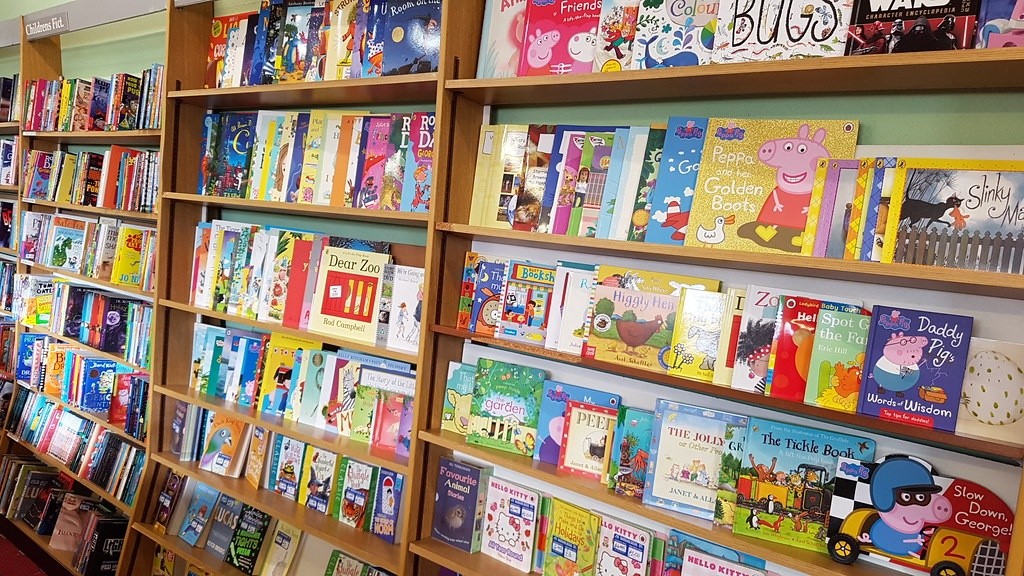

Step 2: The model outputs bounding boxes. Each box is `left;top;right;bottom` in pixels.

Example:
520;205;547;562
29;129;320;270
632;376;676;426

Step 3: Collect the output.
0;0;1024;576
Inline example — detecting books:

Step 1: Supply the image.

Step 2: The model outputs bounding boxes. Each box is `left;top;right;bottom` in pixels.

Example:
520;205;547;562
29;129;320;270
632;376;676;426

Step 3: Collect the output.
169;400;408;545
466;115;1024;278
151;465;400;576
187;217;426;355
457;251;1023;449
189;322;418;459
474;0;1023;81
203;0;442;90
197;106;436;216
426;454;787;575
0;58;165;576
438;356;1015;575
